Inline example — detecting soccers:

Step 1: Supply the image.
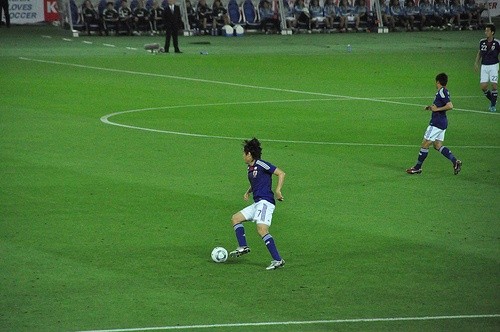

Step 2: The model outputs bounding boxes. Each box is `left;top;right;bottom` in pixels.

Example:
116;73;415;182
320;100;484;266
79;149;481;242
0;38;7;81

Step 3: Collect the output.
210;247;228;263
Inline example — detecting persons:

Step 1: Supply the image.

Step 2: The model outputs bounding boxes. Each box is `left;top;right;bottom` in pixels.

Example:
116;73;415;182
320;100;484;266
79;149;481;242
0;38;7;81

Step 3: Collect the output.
229;137;286;271
0;0;10;27
473;24;500;112
405;73;462;175
80;0;484;36
163;0;185;53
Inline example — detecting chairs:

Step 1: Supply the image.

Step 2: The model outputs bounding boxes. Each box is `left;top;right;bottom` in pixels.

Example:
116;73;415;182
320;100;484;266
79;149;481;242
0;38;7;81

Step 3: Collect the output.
68;0;484;36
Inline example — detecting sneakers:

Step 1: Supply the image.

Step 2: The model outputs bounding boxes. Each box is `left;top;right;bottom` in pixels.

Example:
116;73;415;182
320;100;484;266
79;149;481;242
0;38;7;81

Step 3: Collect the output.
453;160;462;175
266;259;285;270
406;167;422;174
230;246;251;257
489;106;497;112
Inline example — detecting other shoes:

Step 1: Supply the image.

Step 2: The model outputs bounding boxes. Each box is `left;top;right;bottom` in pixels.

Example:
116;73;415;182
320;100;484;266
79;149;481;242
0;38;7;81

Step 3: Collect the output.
388;24;489;32
175;50;181;53
7;25;10;28
277;31;280;34
265;31;269;35
193;28;221;36
165;50;169;52
292;28;370;34
81;31;166;37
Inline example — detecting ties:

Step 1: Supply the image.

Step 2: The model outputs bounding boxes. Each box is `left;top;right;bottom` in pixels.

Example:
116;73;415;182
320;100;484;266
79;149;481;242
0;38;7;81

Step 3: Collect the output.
171;5;172;13
